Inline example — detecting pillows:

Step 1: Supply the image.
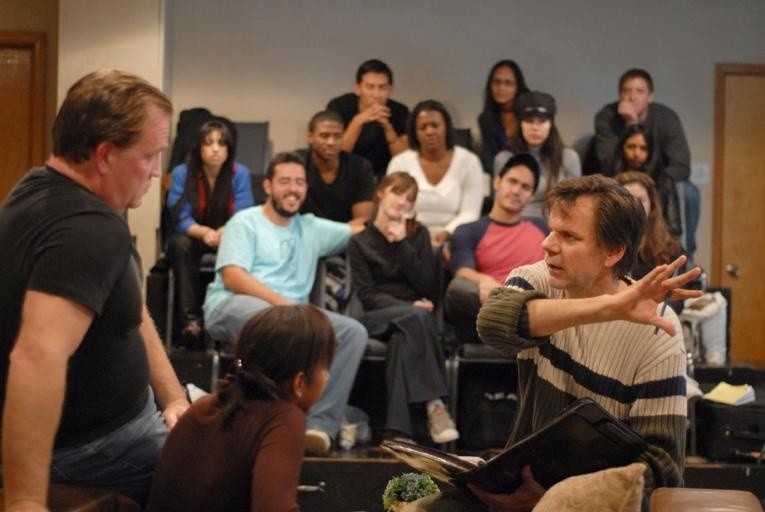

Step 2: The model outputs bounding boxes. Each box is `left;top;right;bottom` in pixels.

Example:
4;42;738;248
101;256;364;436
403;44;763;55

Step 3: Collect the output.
531;463;648;512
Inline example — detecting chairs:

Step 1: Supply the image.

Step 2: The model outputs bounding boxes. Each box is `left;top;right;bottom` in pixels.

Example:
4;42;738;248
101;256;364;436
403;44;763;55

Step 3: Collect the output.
344;249;453;450
204;257;326;393
166;255;216;348
449;354;518;451
234;121;272;205
687;353;697;455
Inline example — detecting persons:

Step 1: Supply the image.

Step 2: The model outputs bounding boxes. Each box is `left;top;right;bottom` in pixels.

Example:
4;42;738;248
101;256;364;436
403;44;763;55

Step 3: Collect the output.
143;301;333;512
467;176;703;512
295;56;693;236
1;69;191;512
614;170;697;320
204;152;368;455
343;171;462;451
165;116;254;339
448;151;548;340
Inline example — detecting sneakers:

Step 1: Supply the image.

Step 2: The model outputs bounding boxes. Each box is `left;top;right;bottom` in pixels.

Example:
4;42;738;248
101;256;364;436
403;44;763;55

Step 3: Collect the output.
172;322;203;350
303;430;330;454
427;406;459;444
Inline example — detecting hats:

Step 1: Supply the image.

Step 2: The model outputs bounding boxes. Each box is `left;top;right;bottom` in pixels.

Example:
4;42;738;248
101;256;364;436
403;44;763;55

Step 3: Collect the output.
514;91;555;119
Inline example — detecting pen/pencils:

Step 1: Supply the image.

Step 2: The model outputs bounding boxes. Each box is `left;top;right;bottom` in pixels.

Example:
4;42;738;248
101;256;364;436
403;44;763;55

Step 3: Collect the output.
655;267;682;337
